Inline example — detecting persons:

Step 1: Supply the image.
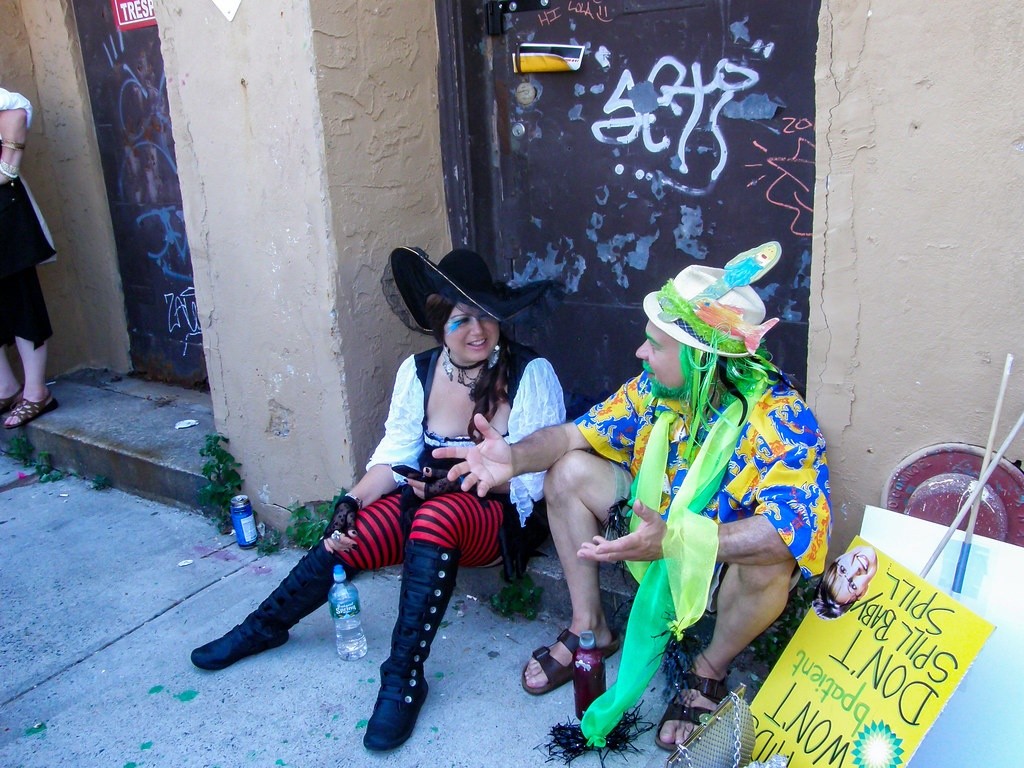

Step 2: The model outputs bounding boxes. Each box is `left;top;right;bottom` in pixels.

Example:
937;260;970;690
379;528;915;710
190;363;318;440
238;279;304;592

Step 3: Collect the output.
190;249;566;751
812;546;879;620
0;87;59;429
432;264;833;751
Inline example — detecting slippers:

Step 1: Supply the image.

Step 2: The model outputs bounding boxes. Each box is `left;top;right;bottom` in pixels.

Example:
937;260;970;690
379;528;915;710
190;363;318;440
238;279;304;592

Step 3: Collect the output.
520;626;621;697
654;667;726;752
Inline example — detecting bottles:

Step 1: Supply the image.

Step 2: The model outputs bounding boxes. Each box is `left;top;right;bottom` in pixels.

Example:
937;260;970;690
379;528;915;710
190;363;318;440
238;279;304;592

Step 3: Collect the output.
572;630;606;721
328;565;368;660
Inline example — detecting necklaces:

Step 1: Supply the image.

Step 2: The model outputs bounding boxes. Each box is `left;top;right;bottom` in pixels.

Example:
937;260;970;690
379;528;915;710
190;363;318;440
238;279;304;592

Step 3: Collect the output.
442;342;487;388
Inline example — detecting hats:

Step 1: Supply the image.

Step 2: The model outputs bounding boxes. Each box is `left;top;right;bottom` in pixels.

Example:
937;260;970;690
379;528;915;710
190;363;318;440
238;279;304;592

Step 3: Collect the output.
642;240;782;358
382;246;566;335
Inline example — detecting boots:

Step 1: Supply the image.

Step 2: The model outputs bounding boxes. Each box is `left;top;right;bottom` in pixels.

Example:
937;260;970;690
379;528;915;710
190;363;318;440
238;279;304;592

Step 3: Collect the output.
190;538;359;671
363;540;463;750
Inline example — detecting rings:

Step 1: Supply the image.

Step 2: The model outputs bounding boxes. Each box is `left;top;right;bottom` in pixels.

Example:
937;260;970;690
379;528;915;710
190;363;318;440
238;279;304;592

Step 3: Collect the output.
331;530;342;542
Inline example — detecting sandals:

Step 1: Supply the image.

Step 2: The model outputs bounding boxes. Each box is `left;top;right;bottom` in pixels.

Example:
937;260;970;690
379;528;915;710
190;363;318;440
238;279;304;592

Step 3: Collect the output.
3;390;60;430
0;384;25;416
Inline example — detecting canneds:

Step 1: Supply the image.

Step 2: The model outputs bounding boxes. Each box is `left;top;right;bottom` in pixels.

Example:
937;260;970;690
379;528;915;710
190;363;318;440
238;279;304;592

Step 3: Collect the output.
230;495;258;549
